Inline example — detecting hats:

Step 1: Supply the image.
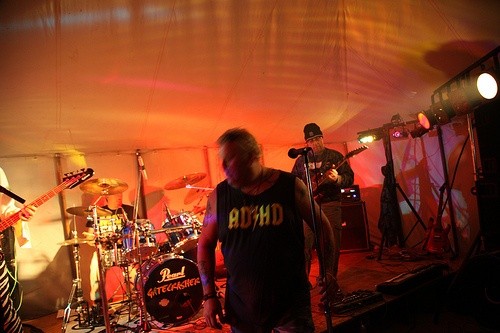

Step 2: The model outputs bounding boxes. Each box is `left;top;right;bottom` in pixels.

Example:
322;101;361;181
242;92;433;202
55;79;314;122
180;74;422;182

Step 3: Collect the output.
303;123;324;141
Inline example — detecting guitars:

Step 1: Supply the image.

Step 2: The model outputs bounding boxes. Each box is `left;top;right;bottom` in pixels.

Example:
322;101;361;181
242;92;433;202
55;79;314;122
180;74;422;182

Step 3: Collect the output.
422;181;451;254
0;168;95;233
305;145;368;201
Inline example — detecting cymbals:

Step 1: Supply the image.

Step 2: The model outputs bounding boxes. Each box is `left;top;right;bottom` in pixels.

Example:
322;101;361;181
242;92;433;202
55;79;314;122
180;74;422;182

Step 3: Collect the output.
65;205;112;218
79;177;128;196
184;184;208;205
57;238;87;246
163;172;207;191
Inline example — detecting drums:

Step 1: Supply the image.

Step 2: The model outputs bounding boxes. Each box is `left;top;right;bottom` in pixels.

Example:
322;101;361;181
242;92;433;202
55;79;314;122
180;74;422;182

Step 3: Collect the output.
132;252;204;327
121;219;157;259
162;211;203;254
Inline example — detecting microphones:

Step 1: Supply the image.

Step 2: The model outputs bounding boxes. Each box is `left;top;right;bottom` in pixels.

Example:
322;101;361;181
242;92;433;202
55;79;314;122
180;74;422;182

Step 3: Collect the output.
136;148;148;180
288;147;312;158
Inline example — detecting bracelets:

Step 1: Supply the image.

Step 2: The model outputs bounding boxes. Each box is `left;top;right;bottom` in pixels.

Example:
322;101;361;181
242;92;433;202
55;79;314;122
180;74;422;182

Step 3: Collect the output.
202;291;217;301
326;271;337;282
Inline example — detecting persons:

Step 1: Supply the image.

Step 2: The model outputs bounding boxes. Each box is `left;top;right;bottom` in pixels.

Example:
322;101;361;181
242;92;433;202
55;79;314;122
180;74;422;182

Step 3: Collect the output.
85;192;141;301
0;167;37;333
196;127;338;333
290;122;354;298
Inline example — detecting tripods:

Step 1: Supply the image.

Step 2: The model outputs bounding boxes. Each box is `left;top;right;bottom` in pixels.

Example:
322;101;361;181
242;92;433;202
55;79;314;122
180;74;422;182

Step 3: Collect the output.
375;136;427;262
427;127;460;258
